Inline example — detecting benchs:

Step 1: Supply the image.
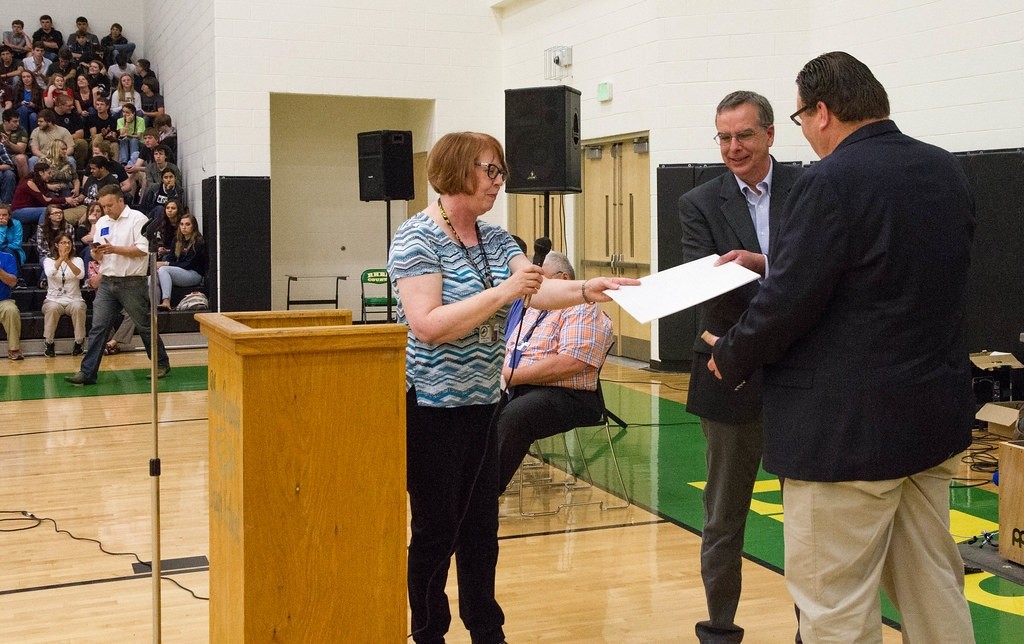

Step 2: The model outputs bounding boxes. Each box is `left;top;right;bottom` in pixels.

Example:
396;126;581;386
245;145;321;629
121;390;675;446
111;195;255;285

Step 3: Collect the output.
0;220;213;341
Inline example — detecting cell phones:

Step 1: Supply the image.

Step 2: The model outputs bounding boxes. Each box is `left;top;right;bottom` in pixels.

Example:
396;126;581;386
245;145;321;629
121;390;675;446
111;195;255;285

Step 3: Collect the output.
92;242;101;248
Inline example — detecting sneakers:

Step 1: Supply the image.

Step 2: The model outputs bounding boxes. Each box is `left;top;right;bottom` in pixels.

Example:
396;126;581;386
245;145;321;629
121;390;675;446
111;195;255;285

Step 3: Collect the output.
72;341;85;355
8;348;24;360
44;339;57;358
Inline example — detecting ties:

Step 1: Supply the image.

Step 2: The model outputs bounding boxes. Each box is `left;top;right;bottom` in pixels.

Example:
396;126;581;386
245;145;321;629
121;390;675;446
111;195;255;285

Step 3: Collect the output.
507;308;550;402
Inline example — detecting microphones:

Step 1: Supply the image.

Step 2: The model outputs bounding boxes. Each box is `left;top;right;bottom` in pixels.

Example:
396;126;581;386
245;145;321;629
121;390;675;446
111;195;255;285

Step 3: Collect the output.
521;237;551;317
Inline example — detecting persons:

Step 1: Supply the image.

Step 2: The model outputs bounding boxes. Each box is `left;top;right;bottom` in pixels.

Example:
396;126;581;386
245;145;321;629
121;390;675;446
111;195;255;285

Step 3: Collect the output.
707;51;979;644
683;90;809;644
0;15;207;360
65;184;171;384
504;234;527;344
386;132;640;644
497;252;614;500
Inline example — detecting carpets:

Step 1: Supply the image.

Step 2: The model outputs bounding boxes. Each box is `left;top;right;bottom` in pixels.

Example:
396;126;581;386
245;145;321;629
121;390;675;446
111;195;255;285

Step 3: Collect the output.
0;364;209;402
957;530;1024;586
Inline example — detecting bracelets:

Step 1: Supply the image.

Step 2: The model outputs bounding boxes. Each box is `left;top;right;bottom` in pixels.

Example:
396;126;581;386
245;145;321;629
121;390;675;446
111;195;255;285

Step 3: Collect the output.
6;73;7;77
582;279;596;305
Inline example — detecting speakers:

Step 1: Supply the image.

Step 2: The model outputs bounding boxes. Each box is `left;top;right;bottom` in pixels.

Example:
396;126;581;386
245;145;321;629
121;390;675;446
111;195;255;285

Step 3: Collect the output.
505;85;582;195
357;129;415;202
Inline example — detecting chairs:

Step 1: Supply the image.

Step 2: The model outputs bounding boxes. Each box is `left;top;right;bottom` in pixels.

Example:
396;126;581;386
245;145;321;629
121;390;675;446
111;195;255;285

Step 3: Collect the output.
361;267;398;325
497;340;630;517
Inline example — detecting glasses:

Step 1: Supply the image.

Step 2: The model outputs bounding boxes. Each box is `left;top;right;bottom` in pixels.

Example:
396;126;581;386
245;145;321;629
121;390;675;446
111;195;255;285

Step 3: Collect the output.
50;211;63;215
475;160;507;182
714;126;771;146
790;97;824;126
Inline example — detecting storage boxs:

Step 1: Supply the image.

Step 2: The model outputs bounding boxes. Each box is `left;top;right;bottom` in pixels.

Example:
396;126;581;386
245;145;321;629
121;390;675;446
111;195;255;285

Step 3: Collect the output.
996;440;1024;568
975;399;1024;441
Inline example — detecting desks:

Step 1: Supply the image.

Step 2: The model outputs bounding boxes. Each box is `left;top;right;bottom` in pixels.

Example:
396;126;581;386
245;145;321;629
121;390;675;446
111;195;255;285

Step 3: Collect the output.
284;274;348;310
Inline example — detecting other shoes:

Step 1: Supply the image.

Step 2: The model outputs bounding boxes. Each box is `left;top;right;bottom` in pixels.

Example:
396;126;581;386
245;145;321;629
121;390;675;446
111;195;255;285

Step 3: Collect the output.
64;371;98;385
157;302;171;310
104;343;120;354
40;280;49;288
15;279;27;289
146;366;169;378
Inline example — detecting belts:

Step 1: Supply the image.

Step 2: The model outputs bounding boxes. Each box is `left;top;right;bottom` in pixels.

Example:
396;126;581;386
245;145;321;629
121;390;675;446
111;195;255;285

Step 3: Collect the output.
103;272;141;283
